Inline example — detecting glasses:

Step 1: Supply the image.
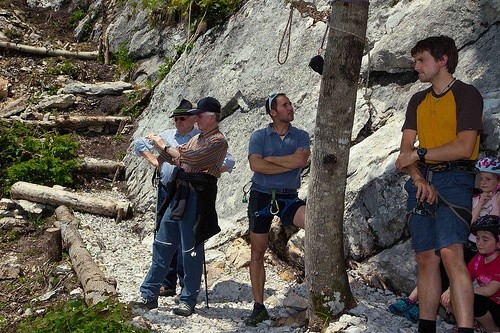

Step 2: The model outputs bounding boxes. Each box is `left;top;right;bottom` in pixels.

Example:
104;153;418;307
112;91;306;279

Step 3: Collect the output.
268;93;278;110
174;115;190;122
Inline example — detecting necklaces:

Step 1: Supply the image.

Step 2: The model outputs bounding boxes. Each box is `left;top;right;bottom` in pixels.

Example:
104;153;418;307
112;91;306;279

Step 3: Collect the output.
272;126;288;136
434;77;456;103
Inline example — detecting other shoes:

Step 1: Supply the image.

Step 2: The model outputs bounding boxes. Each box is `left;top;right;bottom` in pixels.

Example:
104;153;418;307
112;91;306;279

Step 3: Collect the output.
389;298;413;314
451;320;484;333
159;287;176;296
244;302;269;326
175;293;182;303
404;306;419;323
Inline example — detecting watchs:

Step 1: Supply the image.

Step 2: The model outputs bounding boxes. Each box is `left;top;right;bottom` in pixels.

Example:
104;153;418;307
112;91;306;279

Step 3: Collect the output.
164;145;170;151
417;148;427;162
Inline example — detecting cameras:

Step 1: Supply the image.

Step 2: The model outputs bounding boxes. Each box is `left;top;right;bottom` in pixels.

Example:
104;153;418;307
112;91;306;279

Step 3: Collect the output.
148;139;155;145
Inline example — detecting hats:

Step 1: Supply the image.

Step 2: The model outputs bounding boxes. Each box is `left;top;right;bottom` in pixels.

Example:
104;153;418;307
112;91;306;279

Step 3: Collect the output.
187;97;221;114
168;99;192;118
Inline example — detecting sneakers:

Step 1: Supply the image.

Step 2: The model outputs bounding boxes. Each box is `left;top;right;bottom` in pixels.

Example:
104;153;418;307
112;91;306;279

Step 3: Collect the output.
130;296;158;310
173;302;192;316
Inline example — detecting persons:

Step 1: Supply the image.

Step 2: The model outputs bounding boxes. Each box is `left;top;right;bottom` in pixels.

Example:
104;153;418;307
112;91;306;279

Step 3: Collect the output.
388;157;500;324
244;92;311;327
437;215;500;333
134;99;235;297
395;35;483;333
129;97;228;316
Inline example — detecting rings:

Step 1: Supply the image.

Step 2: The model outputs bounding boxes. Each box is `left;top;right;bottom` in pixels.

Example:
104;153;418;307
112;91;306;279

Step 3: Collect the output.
301;149;303;151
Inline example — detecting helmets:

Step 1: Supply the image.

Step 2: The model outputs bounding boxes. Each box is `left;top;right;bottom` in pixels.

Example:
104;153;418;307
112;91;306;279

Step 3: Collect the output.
475;156;500;174
470;214;500;236
